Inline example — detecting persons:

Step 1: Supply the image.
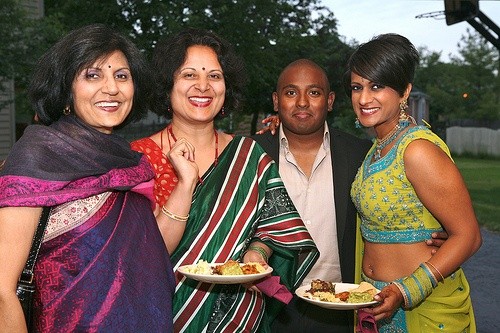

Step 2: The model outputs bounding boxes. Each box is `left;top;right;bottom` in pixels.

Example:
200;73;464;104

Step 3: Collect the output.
125;29;320;333
243;59;374;333
254;33;483;333
0;24;174;333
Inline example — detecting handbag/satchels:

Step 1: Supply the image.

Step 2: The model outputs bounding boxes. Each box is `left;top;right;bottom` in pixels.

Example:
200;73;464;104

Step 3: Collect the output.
16;270;37;333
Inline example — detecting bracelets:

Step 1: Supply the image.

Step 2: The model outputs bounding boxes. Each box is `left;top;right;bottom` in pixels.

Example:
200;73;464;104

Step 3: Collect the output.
391;260;446;310
161;205;190;223
247;246;269;265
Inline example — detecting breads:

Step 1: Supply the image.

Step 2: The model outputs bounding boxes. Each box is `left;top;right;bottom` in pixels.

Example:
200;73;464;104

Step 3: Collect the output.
219;259;245;275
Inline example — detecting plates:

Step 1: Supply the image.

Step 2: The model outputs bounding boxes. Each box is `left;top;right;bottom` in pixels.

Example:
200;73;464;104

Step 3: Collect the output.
176;262;273;284
295;282;382;310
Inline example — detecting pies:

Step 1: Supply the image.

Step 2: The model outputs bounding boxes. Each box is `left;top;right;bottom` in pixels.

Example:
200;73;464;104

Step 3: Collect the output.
349;280;377;300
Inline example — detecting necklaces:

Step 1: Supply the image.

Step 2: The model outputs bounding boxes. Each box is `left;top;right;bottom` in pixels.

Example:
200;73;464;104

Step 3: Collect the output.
167;122;219;188
372;119;410;163
